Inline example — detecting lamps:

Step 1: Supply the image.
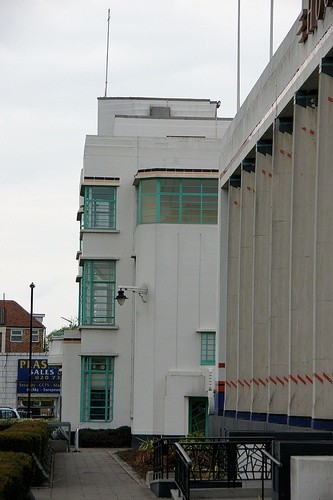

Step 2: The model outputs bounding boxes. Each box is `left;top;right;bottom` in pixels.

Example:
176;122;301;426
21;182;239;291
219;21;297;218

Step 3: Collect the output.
114;284;148;307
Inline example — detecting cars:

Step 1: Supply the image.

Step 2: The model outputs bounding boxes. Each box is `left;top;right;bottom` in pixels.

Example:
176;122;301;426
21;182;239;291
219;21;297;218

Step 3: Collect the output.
0;407;22;419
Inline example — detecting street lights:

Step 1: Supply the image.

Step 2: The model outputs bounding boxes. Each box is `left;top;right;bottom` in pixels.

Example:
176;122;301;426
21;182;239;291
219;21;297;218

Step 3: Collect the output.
28;281;35;420
